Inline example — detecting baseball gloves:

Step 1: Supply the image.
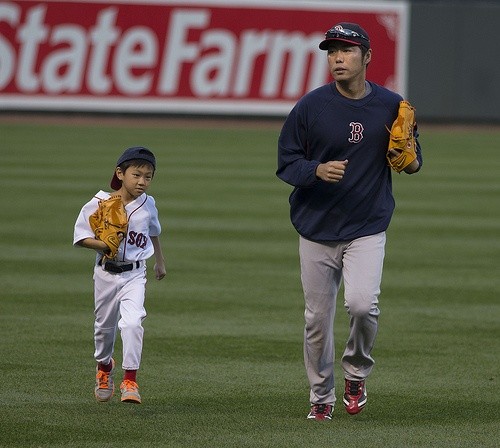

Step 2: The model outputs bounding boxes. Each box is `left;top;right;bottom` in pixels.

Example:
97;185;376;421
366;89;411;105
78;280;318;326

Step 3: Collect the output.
387;101;417;172
89;196;128;258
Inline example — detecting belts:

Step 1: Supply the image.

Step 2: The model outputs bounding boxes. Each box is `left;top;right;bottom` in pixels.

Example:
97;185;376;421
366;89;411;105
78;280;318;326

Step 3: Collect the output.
98;259;140;272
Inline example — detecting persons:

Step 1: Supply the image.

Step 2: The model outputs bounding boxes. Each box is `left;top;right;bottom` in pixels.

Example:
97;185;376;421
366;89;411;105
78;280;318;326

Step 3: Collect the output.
73;147;167;405
276;23;422;418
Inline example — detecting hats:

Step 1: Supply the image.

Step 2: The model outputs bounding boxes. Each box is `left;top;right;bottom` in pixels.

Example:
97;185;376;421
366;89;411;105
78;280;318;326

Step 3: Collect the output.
111;146;156;190
319;22;370;49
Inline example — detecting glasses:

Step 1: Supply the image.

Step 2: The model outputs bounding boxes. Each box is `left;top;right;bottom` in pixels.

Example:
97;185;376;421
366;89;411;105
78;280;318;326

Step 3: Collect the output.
325;29;369;42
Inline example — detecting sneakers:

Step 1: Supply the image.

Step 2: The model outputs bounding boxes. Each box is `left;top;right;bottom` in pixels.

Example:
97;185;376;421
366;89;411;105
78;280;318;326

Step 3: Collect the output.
307;404;334;420
120;379;142;404
95;357;115;402
343;378;367;415
